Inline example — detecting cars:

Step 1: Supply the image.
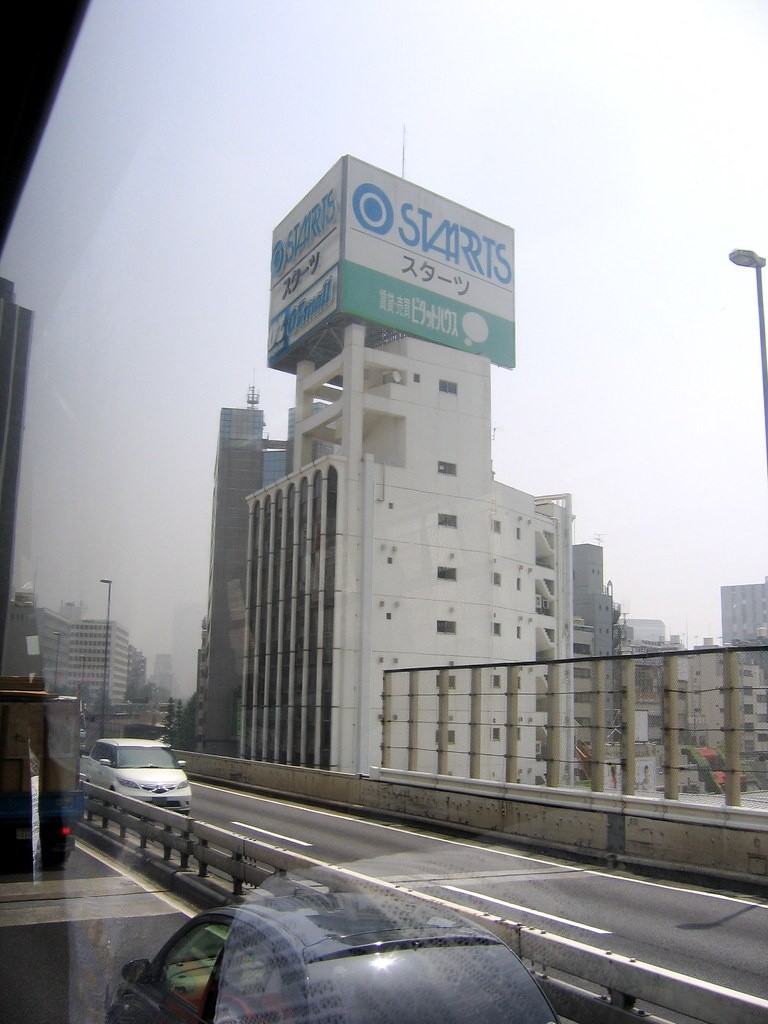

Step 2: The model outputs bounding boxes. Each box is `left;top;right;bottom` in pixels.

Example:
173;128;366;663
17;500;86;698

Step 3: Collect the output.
106;884;564;1024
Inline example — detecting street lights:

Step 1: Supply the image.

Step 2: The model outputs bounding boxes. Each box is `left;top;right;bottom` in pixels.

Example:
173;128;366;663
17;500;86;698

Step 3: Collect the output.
99;577;114;737
729;248;768;482
80;655;86;685
105;668;111;706
52;632;62;688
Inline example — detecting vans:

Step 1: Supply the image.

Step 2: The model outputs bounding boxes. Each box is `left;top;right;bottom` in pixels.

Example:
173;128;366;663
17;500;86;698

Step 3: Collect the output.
86;738;192;816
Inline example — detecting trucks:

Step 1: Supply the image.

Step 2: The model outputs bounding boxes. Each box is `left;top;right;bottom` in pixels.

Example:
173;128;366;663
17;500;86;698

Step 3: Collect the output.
0;673;84;870
